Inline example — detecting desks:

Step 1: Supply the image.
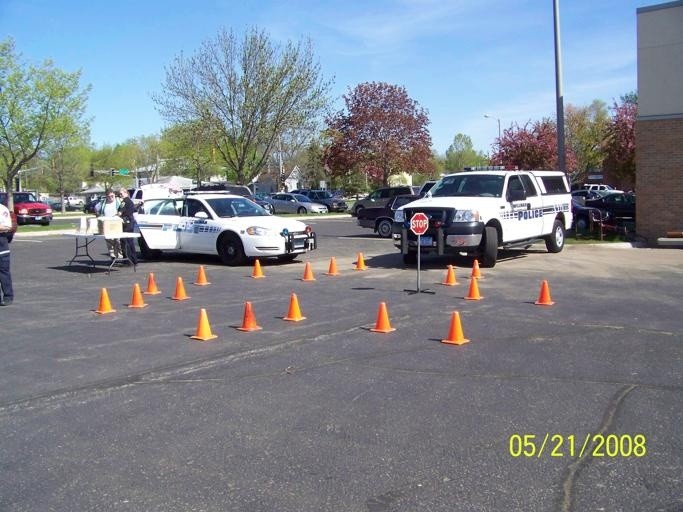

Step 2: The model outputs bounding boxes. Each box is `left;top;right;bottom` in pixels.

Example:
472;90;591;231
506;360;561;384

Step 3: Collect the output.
62;232;141;277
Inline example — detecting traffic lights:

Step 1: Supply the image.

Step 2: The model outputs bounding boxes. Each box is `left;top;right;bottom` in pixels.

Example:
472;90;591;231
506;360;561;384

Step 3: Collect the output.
110;169;115;177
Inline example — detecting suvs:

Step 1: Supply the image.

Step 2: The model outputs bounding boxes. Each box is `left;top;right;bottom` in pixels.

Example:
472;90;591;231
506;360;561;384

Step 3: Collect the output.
297;189;348;212
195;184;257;204
64;196;83;205
0;191;53;225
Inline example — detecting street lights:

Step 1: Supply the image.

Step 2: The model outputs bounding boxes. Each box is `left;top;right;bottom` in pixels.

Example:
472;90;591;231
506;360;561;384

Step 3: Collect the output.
483;113;501;163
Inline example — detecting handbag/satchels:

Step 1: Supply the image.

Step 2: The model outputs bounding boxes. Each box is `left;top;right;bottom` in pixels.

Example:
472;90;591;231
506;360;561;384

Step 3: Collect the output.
0;232;13;243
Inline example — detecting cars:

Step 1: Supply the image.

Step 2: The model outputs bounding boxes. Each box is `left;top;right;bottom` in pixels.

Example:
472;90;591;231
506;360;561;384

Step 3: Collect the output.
83;188;142;214
572;182;636;233
130;183;317;265
53;203;80;211
352;181;436;237
264;192;328;214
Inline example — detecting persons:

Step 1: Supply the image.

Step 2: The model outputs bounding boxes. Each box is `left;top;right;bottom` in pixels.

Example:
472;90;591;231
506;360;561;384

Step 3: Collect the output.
117;188;138;264
95;190;144;258
0;204;14;305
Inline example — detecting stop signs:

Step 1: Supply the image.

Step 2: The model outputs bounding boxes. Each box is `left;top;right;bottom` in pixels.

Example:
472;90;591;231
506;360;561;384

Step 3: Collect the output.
410;212;429;236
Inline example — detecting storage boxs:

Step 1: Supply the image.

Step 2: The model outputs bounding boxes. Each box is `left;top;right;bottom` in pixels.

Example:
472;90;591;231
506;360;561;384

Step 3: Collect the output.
98;219;122;234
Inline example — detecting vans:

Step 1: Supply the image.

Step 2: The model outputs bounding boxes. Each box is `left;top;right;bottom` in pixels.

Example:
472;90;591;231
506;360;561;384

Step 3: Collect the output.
391;170;575;268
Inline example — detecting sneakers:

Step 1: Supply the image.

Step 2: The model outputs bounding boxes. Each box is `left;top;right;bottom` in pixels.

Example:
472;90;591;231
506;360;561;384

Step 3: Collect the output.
0;297;12;306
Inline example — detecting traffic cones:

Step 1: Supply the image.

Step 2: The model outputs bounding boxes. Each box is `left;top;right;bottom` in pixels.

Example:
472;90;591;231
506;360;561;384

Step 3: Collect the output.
535;279;555;306
302;262;314;280
236;302;262;331
370;302;396;333
126;283;148;308
170;276;190;300
443;265;460;285
250;259;264;279
470;259;483;279
354;252;366;270
194;266;210;286
464;278;480;300
325;256;339;275
440;311;470;345
190;307;218;342
144;273;161;295
94;288;116;316
281;292;306;322
